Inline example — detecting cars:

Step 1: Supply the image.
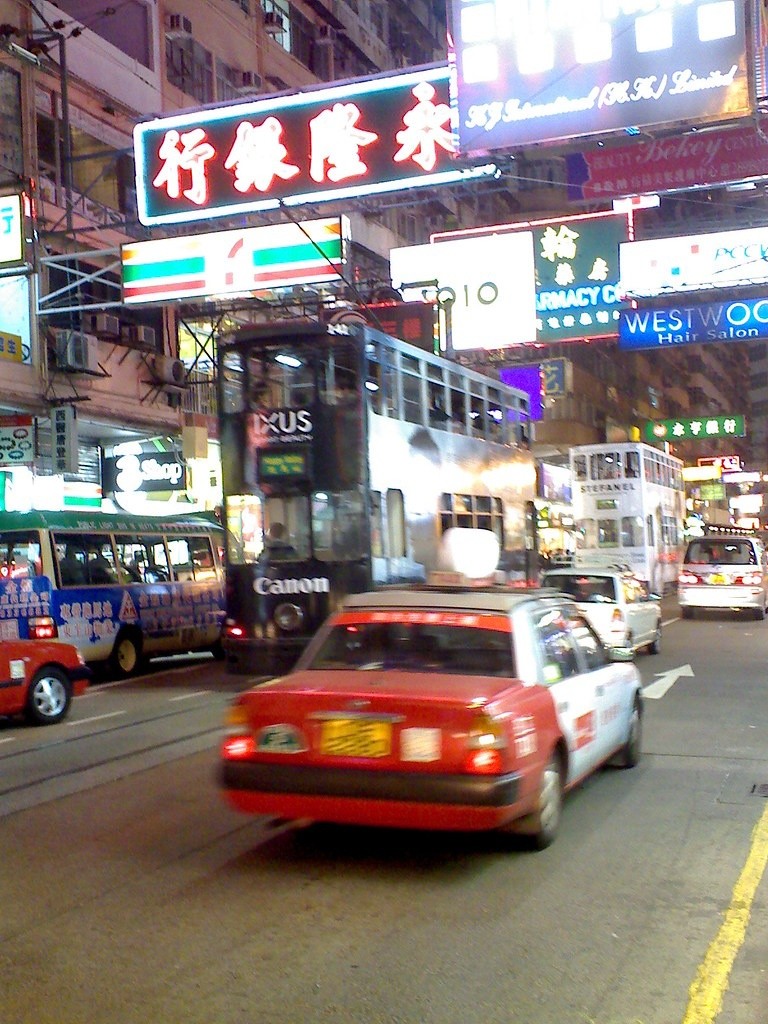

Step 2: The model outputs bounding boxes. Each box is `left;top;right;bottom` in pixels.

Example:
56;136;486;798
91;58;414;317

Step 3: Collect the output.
0;635;93;729
678;535;768;621
205;571;655;852
535;566;664;657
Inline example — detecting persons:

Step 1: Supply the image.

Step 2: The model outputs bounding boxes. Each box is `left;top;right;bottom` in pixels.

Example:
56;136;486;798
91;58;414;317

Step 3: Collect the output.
258;521;299;561
539;542;575;569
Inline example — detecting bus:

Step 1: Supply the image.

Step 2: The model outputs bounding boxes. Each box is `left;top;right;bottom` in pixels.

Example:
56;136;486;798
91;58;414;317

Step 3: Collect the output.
569;442;688;596
1;507;246;676
209;322;537;677
